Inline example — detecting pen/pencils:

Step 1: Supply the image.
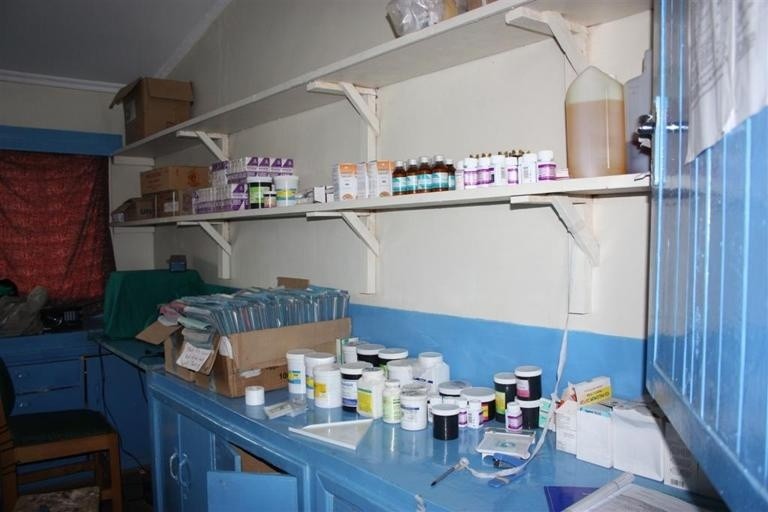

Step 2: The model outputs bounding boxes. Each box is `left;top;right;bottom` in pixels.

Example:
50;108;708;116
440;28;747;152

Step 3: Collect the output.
430;465;458;488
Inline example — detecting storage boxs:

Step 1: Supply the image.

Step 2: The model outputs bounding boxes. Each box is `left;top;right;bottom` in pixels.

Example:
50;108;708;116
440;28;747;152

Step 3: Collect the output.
135;315;352;399
105;76;212;223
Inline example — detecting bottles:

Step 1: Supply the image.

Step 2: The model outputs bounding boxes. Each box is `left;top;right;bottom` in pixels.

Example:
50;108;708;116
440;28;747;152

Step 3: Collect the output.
246;176;298;208
391;149;565;195
286;341;541;442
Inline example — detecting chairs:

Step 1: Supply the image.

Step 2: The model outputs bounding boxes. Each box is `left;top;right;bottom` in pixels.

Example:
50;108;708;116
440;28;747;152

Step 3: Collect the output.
0;357;123;511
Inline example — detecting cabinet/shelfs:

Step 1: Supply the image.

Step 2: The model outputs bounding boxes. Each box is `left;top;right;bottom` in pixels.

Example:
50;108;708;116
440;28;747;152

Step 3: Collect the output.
0;333;105;497
147;392;301;512
107;1;658;318
644;0;767;512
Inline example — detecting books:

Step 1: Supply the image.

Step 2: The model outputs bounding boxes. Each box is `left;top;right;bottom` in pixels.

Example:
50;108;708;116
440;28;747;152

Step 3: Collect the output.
560;470;712;512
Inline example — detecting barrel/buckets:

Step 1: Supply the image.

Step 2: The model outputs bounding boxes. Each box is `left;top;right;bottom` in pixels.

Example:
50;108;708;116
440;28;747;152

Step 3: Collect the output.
565;66;626;176
624;49;652;172
